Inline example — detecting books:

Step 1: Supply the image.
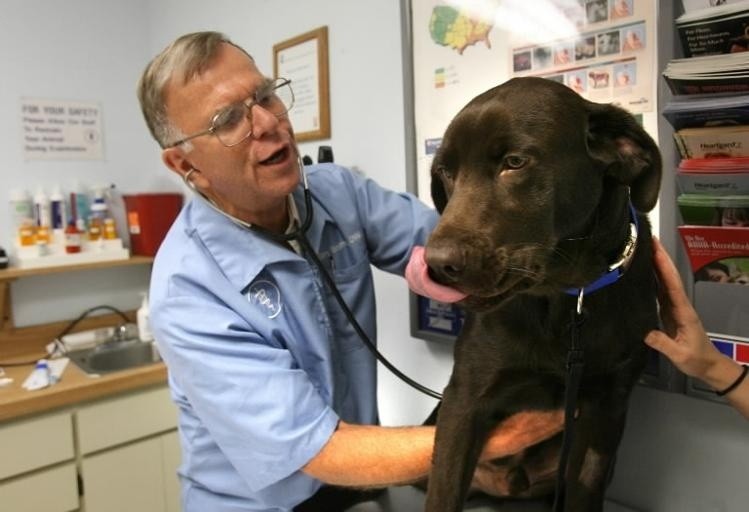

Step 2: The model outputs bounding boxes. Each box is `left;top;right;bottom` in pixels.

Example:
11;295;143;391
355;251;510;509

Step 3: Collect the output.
661;1;749;288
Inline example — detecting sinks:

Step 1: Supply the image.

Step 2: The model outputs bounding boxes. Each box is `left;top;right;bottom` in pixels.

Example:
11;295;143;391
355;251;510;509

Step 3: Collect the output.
63;335;166;378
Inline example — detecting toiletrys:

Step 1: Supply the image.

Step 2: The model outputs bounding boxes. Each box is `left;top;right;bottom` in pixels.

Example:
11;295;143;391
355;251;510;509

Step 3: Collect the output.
134;287;155;342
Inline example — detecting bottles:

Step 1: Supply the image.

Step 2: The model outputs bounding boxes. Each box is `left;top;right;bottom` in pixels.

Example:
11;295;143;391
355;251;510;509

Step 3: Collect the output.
10;186;116;254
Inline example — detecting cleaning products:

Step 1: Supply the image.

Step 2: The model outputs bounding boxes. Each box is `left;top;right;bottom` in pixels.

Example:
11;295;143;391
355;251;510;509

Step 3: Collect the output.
88;179;117;222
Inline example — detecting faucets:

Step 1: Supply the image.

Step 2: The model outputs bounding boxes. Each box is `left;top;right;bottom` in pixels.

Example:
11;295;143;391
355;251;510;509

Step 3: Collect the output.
110;322;131;342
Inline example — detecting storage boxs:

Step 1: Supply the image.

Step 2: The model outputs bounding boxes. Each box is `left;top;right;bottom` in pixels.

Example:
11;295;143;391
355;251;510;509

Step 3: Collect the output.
120;191;182;256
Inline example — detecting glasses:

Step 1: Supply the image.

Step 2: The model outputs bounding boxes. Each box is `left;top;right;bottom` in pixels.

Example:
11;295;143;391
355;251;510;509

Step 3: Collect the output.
157;74;297;153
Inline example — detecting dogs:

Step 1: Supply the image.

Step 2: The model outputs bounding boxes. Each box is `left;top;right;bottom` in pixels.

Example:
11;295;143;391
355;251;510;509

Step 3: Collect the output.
423;77;673;511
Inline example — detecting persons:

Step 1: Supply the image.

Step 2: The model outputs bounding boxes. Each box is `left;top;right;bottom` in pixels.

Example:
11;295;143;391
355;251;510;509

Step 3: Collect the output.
135;30;568;512
640;234;749;420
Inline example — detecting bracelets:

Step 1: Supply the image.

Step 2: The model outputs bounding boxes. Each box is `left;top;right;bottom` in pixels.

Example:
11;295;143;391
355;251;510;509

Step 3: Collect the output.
711;364;749;396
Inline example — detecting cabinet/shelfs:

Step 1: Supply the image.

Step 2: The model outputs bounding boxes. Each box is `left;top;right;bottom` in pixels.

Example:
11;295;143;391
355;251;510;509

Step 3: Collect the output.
0;379;184;512
